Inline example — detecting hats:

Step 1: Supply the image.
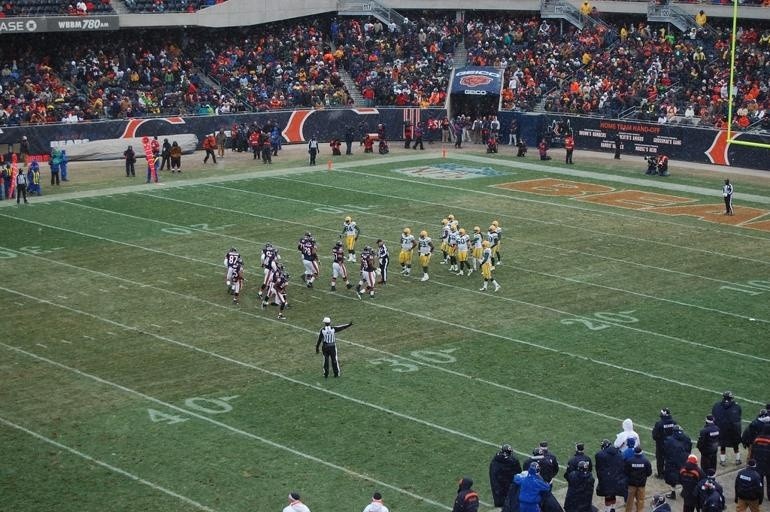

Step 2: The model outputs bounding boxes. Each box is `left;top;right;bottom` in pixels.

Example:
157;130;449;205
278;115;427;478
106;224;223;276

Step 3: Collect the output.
759;409;766;415
376;239;381;243
635;446;641;453
688;455;697;463
540;441;547;448
627;438;634;444
322;318;330;323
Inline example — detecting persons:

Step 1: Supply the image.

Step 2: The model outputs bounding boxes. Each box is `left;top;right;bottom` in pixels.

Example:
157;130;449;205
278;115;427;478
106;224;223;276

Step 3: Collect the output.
650;407;678;481
361;492;392;512
734;457;764;511
283;490;311;512
711;390;744;468
452;478;480;512
222;212;505;321
721;178;736;216
741;402;770;504
694;414;721;477
0;0;769;204
488;419;728;512
314;316;355;378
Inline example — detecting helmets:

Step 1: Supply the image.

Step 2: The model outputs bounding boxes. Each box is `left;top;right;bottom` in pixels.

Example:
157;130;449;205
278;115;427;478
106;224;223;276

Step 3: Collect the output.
482;241;490;247
442;215;465;235
602;439;610;448
578;461;587;471
653;494;665;505
489;221;497;232
475;226;480;233
419;230;427;237
502;444;513;453
530;462;540;472
404;228;411;234
723;391;732;397
534;448;543;455
345;216;352;222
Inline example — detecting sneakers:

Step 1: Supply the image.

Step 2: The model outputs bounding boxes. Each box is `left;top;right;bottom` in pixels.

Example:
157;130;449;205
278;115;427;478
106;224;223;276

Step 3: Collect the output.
479;287;487;291
401;267;406;273
666;494;675;499
467;269;472;275
494;286;500;291
456;272;463;276
421;275;429;281
